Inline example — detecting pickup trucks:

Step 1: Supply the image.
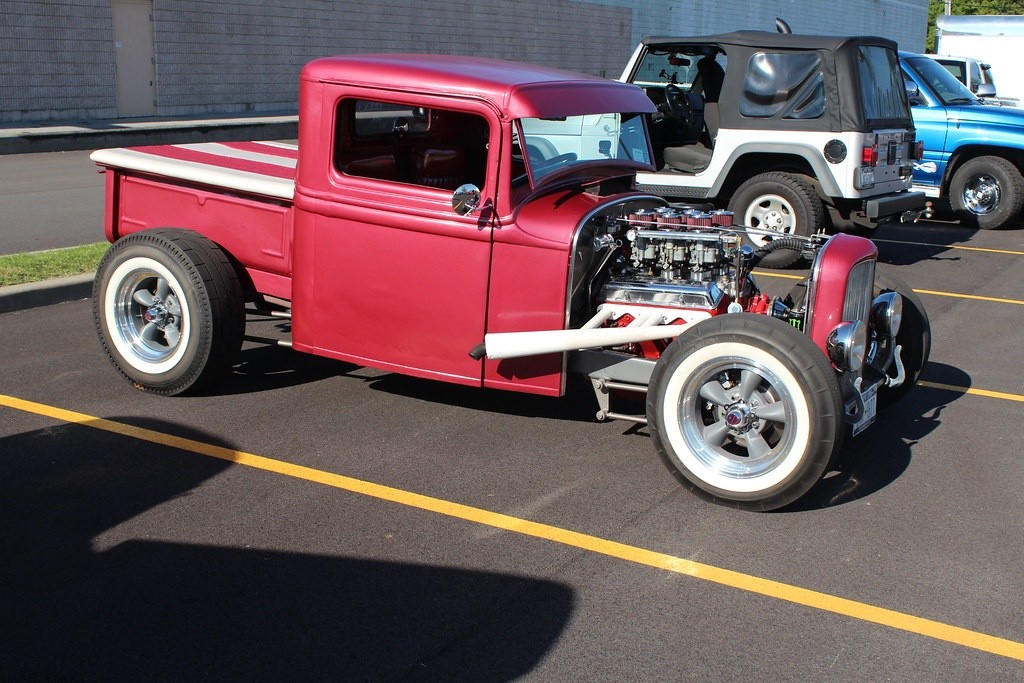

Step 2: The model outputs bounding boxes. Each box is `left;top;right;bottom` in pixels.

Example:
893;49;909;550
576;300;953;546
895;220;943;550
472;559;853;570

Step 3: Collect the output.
90;55;931;513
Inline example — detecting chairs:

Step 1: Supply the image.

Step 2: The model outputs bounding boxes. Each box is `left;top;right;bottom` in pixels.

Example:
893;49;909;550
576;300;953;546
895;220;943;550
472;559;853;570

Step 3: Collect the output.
411;142;465;188
664;57;726;173
343;152;398;178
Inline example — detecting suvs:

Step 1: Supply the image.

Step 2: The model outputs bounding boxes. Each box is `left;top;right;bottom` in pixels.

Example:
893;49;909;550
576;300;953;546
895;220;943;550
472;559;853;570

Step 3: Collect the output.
922;54;1020;108
510;16;935;269
893;51;1024;229
